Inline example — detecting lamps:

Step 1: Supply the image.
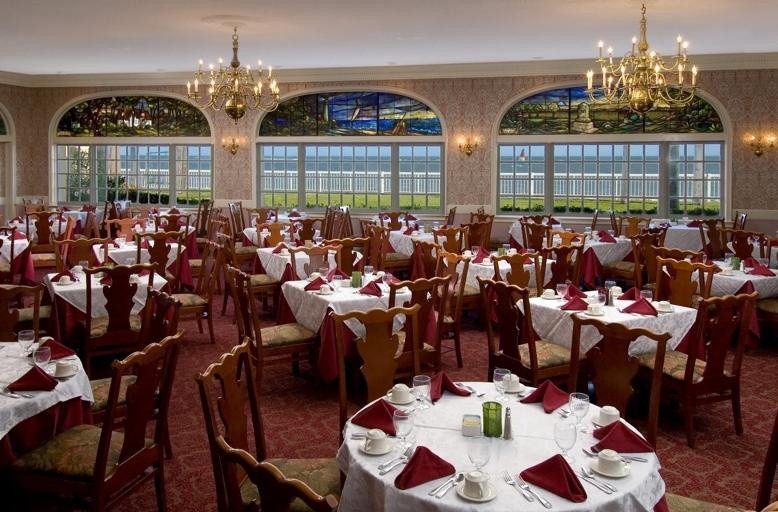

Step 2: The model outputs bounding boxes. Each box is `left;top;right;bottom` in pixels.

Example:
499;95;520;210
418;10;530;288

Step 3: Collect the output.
186;29;282;122
458;136;481;156
221;137;240;155
743;131;777;158
584;3;702;116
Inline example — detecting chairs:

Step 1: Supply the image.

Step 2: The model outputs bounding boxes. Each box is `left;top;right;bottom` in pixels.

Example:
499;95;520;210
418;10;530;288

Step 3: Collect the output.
88;375;174;459
214;435;341;509
195;337;346;506
9;325;184;510
1;196;776;393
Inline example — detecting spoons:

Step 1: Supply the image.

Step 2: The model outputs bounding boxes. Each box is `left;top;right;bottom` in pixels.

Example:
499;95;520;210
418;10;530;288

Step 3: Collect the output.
582;448;649;463
581;466;618;492
467;384;486;398
436;474;466;499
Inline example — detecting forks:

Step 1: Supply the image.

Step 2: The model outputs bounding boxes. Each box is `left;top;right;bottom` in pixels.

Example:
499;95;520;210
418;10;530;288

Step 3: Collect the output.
379;447;415;477
512;473;552;509
500;470;532;503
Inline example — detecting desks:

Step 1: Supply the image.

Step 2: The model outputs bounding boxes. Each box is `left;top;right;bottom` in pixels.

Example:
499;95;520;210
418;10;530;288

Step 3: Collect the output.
0;341;89;473
343;382;658;509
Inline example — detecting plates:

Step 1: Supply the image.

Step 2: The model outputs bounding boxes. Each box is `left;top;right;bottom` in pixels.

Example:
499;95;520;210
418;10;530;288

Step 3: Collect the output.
587;459;631;478
455;482;496;501
45;364;76;377
593;415;625;427
390;390;414;404
359;441;392;455
503;382;526;393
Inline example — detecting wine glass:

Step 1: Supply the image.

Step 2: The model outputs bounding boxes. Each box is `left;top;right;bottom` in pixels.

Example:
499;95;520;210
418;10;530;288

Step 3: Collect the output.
494;367;512;401
554;392;590;463
17;330;36;363
753;232;760;247
412;375;432;410
394;409;414;446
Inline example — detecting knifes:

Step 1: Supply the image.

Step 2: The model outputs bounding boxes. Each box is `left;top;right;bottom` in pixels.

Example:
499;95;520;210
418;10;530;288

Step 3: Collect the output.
572;471;612;494
427;472;465;496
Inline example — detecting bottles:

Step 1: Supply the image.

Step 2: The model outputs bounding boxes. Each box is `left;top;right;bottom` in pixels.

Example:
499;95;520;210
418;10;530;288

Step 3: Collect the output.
503;406;514;441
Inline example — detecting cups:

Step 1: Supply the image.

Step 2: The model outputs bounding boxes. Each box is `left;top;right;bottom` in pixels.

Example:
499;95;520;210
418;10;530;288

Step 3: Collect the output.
466;435;492;470
32;346;52;370
55;361;79;375
408;221;440;235
502;372;520;389
482;402;504;438
564;225;628;241
280;237;324;256
363;428;387;451
58;258;141;285
597;451;628;474
263;228;268;233
463;242;543;266
541;280;672;316
308;264;405;295
598;407;619;424
683;253;740;272
386;383;410;401
464;470;488;500
